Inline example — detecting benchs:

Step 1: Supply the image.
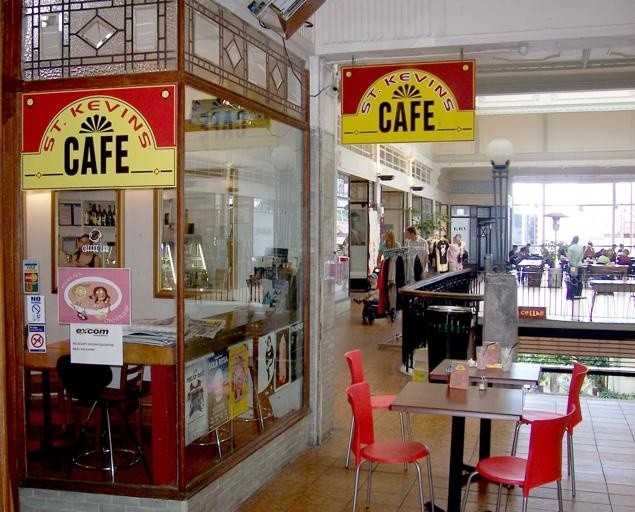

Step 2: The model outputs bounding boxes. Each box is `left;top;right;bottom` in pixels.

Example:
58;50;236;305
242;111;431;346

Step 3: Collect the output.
517;335;635;376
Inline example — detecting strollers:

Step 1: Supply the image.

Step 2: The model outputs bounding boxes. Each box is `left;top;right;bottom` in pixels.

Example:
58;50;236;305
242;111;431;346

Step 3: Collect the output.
363;267;395;324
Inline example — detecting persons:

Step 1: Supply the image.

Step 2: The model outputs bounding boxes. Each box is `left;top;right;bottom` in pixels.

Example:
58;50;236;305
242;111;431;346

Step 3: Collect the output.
353;273;380;305
561;236;584;296
539;245;549;256
448;237;461;271
72;234;103;269
520;244;530;257
405;226;429;280
508;245;518;264
583;242;632;266
376;229;401;265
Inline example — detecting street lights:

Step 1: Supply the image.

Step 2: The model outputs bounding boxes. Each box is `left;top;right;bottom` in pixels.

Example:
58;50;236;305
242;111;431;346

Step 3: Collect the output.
543;212;570;252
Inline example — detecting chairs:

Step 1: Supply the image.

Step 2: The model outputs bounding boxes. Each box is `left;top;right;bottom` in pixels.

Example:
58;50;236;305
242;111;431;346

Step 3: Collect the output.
346;383;435;512
24;327;72;431
511;363;588;497
462;405;577;511
99;363;145;448
343;348;412;471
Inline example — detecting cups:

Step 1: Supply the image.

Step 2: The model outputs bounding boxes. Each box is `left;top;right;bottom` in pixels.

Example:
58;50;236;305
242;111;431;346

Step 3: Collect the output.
476;347;488;370
500;346;513;372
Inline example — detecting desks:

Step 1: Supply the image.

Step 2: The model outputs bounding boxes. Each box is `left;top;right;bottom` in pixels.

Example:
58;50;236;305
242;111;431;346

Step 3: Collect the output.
518;260;543;285
590;279;635;322
123;309;296;485
25;339;98;472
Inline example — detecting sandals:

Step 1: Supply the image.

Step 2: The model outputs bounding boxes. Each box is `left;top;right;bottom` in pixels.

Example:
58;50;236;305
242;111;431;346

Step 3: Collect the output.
353;298;361;304
363;299;369;305
